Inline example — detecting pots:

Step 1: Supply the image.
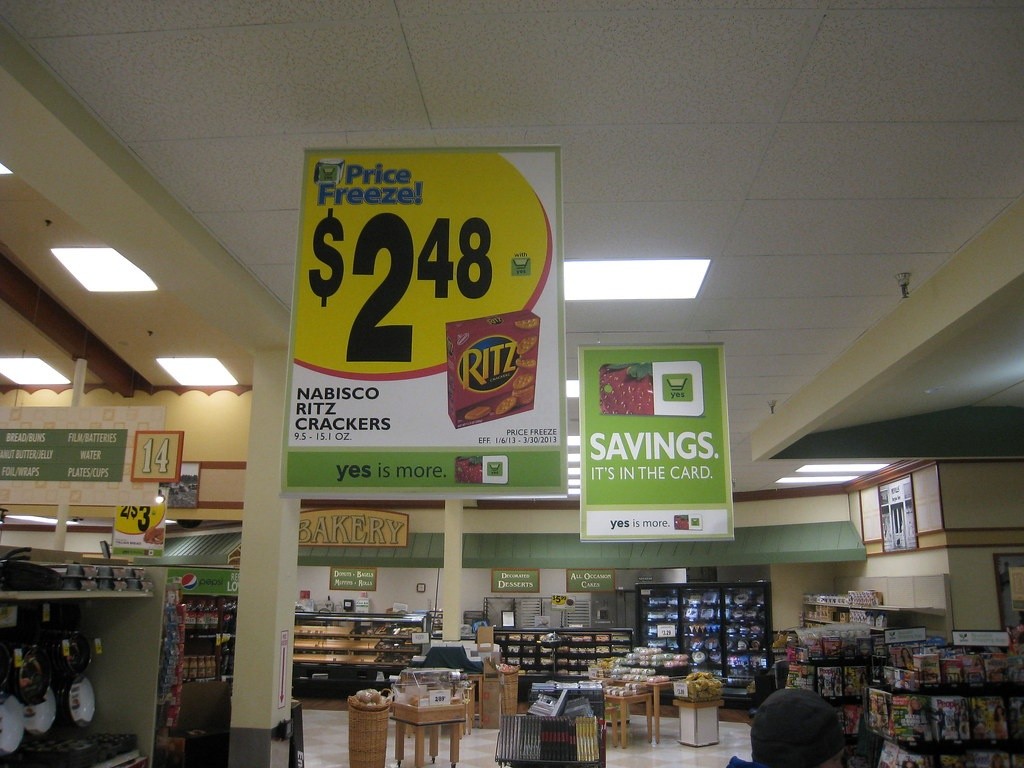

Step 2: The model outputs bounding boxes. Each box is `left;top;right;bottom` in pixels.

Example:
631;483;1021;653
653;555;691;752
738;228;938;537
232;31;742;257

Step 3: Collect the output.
0;622;95;755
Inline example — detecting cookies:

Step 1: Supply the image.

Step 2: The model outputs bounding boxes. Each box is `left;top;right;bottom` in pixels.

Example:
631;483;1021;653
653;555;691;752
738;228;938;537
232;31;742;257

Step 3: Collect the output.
464;320;538;419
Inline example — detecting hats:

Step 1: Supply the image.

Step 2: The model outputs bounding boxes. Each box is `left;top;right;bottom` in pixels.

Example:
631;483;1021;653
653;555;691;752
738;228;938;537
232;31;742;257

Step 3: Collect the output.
750;688;845;768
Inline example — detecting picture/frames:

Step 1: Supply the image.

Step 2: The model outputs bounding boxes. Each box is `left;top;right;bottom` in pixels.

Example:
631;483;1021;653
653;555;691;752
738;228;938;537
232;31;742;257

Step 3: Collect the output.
877;472;919;552
131;430;185;484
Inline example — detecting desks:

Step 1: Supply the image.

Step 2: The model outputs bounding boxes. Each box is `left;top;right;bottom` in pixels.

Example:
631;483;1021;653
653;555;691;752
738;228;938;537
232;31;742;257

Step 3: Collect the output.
590;675;678;749
389;672;484;768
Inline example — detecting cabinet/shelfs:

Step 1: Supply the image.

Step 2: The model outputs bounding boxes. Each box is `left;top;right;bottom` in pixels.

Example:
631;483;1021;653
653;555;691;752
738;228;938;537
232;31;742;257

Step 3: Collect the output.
0;559;168;768
472;627;634;705
635;579;772;711
292;611;431;694
794;573;1024;768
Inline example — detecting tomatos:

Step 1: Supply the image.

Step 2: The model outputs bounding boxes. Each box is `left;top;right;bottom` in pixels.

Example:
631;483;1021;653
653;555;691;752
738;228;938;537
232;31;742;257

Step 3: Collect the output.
457;460;482;482
600;366;654;416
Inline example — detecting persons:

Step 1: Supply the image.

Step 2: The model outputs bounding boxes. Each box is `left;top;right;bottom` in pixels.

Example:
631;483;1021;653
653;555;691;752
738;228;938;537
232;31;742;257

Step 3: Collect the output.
726;688;845;768
900;648;1008;768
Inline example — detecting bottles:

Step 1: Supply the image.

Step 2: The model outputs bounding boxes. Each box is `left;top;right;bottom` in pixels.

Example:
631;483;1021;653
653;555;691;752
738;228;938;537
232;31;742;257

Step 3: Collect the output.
221;641;234;675
183;655;216;678
185;600;237;633
